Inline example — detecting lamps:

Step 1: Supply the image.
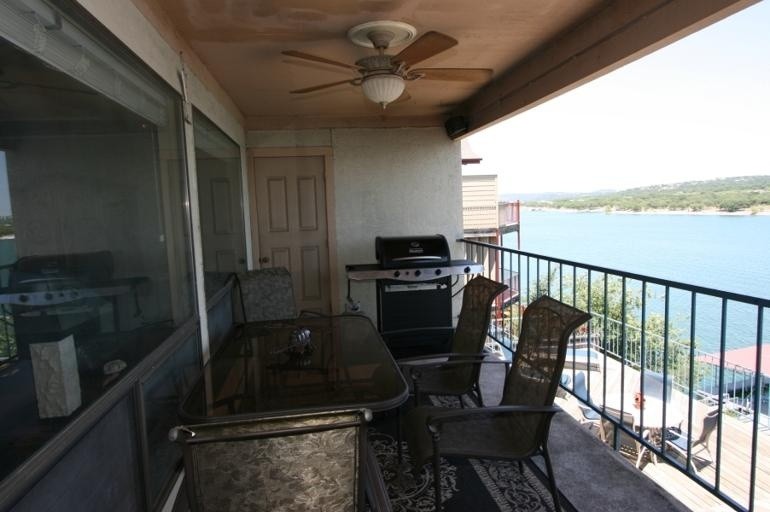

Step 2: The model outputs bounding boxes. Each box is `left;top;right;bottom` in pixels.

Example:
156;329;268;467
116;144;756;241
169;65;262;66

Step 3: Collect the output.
353;71;409;111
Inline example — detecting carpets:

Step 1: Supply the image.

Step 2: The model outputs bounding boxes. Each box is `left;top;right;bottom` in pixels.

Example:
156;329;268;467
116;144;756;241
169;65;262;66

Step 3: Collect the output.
355;353;576;510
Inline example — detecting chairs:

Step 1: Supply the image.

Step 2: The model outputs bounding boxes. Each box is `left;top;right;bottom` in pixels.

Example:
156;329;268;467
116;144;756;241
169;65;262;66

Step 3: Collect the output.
382;274;509;466
409;294;593;512
226;265;340;399
572;371;722;481
168;404;392;512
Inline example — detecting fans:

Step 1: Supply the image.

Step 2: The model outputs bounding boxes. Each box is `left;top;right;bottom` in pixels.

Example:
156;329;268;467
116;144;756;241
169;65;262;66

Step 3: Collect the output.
279;19;496;102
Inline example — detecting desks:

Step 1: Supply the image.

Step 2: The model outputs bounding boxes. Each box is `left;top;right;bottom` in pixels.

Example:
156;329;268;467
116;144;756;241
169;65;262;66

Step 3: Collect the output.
180;312;412;469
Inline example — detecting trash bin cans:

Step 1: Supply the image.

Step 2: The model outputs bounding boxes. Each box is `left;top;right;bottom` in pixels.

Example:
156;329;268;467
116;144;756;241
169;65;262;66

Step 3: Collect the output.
28;334;83;419
634;394;646;409
